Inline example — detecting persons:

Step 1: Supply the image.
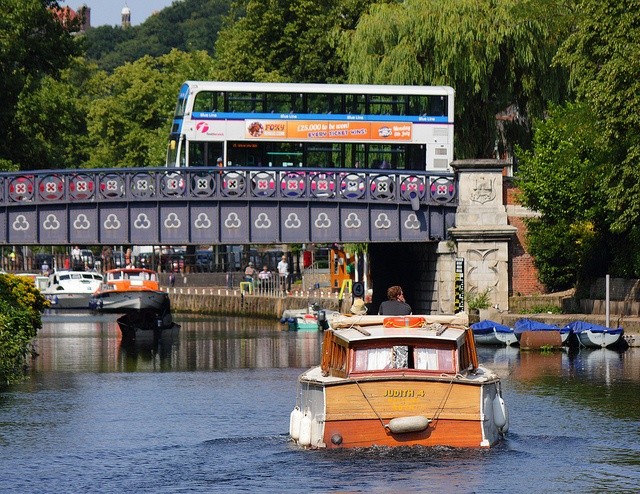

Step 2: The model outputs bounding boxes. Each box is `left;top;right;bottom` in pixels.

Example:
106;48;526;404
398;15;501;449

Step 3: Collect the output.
42;261;50;277
258;266;273;293
71;246;83;267
168;271;175;288
126;262;136;269
244;262;257;294
277;255;292;296
138;258;150;269
378;285;413;316
7;249;16;268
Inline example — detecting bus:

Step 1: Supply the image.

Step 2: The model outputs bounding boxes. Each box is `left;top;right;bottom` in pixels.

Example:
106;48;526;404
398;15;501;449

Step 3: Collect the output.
164;79;455;201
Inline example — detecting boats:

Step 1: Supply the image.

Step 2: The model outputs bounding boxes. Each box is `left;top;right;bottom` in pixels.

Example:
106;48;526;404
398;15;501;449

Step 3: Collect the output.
42;271;104;308
117;310;181;338
287;312;509;451
282;309;320;330
471;319;517;345
573;321;624;348
90;267;170;312
518;322;572;347
